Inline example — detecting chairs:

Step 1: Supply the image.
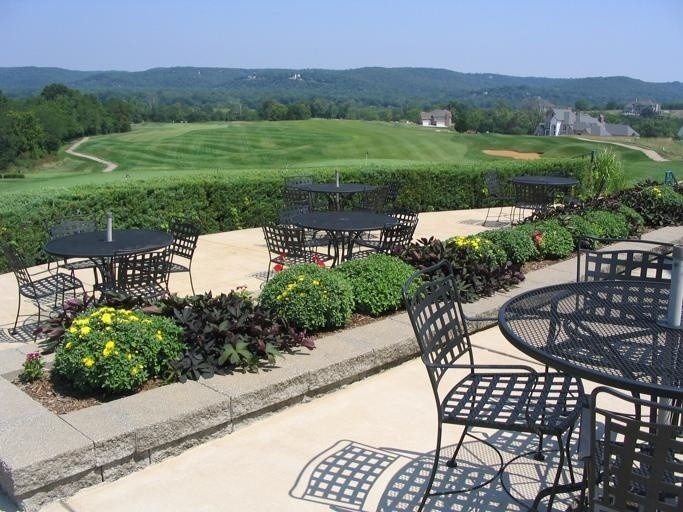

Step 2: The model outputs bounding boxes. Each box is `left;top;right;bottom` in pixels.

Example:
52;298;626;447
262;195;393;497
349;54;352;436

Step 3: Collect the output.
5;217;201;344
288;439;565;512
263;175;419;282
569;385;682;512
0;319;52;344
482;167;609;226
535;238;682;462
400;243;600;512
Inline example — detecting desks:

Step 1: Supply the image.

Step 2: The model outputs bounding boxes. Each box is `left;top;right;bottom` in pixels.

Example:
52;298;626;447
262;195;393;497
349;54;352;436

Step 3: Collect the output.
499;279;682;512
